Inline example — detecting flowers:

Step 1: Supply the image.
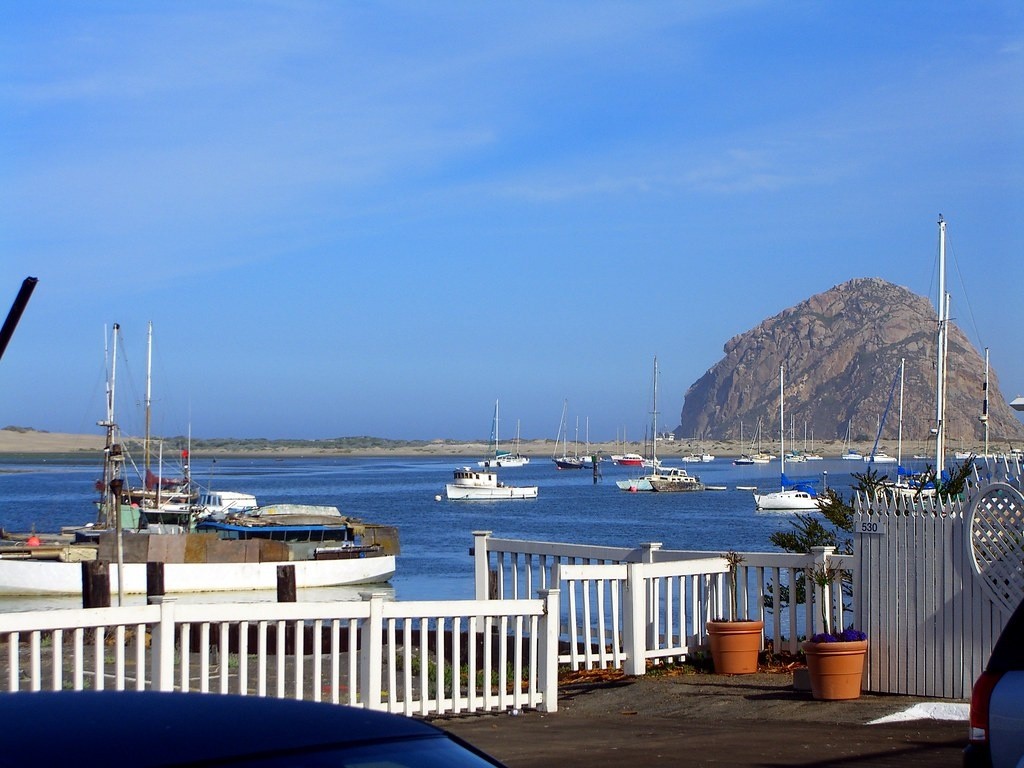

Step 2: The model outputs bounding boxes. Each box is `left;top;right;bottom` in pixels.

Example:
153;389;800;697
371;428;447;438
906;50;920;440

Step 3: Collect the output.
805;556;867;643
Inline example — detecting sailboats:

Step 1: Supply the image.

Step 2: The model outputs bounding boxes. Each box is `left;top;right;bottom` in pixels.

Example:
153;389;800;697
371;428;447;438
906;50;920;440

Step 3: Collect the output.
879;213;1024;501
478;396;530;468
840;419;863;459
551;354;707;491
863;413;899;464
751;364;836;509
734;412;824;465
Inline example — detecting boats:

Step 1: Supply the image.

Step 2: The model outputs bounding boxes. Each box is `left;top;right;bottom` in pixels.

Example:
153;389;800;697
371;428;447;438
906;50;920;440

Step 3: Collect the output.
446;466;540;500
1;318;401;601
682;451;716;462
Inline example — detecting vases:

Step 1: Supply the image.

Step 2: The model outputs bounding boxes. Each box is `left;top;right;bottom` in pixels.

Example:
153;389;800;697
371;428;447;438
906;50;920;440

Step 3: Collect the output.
706;617;764;675
800;640;868;700
